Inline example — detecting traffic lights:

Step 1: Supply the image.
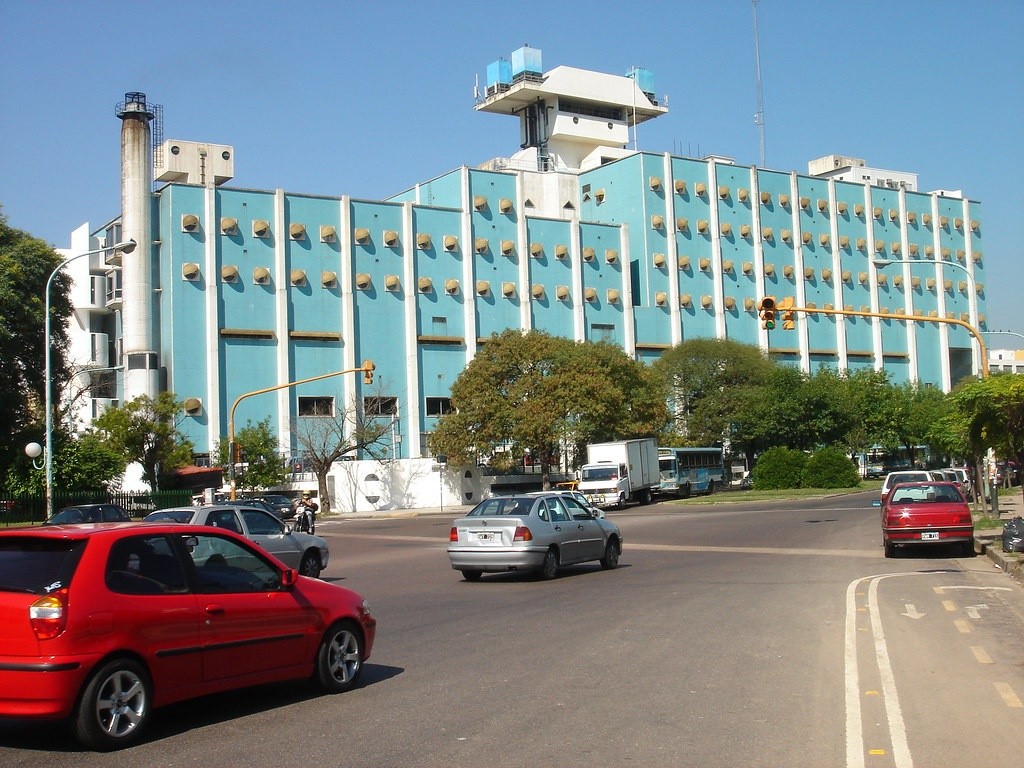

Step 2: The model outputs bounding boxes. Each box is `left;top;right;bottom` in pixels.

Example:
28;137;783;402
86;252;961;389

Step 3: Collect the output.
365;360;376;384
756;296;795;331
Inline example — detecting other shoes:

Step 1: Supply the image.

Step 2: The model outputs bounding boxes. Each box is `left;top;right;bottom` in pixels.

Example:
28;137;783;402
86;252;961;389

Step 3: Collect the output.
308;527;313;533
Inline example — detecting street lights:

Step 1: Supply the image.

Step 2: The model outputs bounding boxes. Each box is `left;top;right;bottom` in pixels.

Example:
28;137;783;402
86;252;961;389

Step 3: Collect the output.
871;258;980;376
25;240;138;523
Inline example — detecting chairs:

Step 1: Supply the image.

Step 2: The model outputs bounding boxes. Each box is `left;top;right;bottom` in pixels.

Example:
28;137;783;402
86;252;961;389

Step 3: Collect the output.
511;507;528;515
543;511;559;520
141;554;183;592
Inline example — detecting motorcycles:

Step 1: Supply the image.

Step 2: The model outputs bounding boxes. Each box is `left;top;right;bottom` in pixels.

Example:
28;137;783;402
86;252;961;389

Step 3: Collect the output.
292;505;316;536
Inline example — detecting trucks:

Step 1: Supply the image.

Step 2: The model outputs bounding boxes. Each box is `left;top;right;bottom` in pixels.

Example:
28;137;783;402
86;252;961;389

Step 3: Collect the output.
573;438;662;511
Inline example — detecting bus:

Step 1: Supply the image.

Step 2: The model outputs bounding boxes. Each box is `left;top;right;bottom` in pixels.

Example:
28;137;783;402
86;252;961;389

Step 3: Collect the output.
861;444;928;471
649;447;725;498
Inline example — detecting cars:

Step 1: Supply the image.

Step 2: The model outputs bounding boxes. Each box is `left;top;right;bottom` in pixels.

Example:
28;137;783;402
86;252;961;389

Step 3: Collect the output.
253;495;296;519
871;480;976;556
879;462;1021;507
512;490;607;520
548;480;579;492
866;462;888;477
216;499;285;535
0;520;376;753
446;493;623;580
140;503;330;585
35;503;133;549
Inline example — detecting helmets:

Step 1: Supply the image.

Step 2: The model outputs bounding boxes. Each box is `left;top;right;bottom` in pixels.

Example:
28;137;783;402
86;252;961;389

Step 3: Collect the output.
302;489;311;499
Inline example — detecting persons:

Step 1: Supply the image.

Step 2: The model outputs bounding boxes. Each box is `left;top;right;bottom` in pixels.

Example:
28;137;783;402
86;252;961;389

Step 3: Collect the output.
293;490;318;534
197;492;205;506
